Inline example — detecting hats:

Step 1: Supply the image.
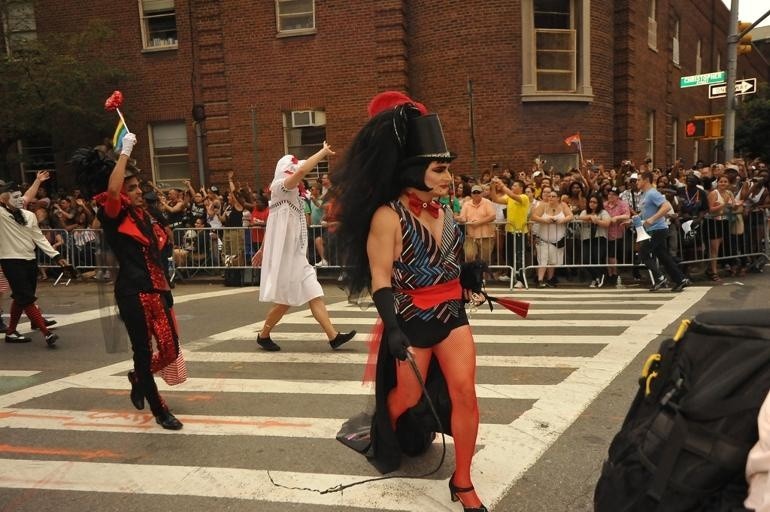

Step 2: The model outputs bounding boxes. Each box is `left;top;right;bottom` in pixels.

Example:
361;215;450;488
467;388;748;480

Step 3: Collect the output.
532;171;542;178
471;185;483;192
606;186;620;197
724;164;740;176
629;172;638;180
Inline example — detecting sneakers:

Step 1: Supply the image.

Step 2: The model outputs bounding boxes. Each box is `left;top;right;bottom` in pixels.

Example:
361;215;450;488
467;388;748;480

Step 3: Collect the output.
5;330;32;343
338;271;347;282
650;274;668;291
586;273;622;288
672;274;690;292
44;331;59;346
313;260;328;266
498;274;560;288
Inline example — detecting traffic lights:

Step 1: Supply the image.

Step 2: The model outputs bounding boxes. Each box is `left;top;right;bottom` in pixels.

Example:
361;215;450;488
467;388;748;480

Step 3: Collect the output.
711;118;722;137
737;19;753;56
686;119;705;138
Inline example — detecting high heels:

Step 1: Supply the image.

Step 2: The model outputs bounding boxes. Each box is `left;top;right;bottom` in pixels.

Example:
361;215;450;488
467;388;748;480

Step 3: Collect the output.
448;470;489;512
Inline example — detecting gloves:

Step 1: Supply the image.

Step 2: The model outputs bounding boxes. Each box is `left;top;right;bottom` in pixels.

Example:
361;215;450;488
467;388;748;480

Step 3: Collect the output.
370;286;410;361
119;133;138;159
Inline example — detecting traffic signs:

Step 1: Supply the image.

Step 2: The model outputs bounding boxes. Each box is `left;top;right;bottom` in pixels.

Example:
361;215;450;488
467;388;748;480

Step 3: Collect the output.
708;78;756;99
680;71;725;88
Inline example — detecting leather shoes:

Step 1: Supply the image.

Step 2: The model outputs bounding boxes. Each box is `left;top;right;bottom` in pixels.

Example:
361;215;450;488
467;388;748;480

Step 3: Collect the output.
329;329;356;349
128;369;146;410
256;333;282;351
0;317;11;333
156;413;183;430
31;317;57;330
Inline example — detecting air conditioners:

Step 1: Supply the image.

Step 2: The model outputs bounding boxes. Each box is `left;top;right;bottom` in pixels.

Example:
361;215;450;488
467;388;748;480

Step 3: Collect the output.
291;110;315;128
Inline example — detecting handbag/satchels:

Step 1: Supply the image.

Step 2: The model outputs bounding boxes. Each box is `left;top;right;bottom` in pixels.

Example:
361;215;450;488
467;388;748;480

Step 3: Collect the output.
552;235;565;249
152;339;187;387
727;212;745;235
459;259;496;295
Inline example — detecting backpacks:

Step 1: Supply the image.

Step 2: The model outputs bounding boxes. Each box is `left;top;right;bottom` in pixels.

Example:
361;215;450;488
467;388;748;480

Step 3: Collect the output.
594;308;770;512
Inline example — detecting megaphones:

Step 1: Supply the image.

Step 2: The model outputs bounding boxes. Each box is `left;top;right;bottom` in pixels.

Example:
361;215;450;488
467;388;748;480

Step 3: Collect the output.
632;215;652;243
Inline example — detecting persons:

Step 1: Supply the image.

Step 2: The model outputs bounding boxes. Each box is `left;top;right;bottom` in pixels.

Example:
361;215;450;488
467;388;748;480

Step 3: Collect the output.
327;156;488;512
90;131;183;430
256;140;357;351
1;154;769;292
1;170;59;334
0;178;69;347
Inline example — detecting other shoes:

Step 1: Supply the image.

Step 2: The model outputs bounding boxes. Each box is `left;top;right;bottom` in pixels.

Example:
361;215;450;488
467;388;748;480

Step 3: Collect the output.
94;270;111;280
706;264;764;282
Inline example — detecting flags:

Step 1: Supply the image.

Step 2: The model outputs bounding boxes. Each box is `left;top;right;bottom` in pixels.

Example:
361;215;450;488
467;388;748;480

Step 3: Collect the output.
113;118;129;151
563;132;580;147
